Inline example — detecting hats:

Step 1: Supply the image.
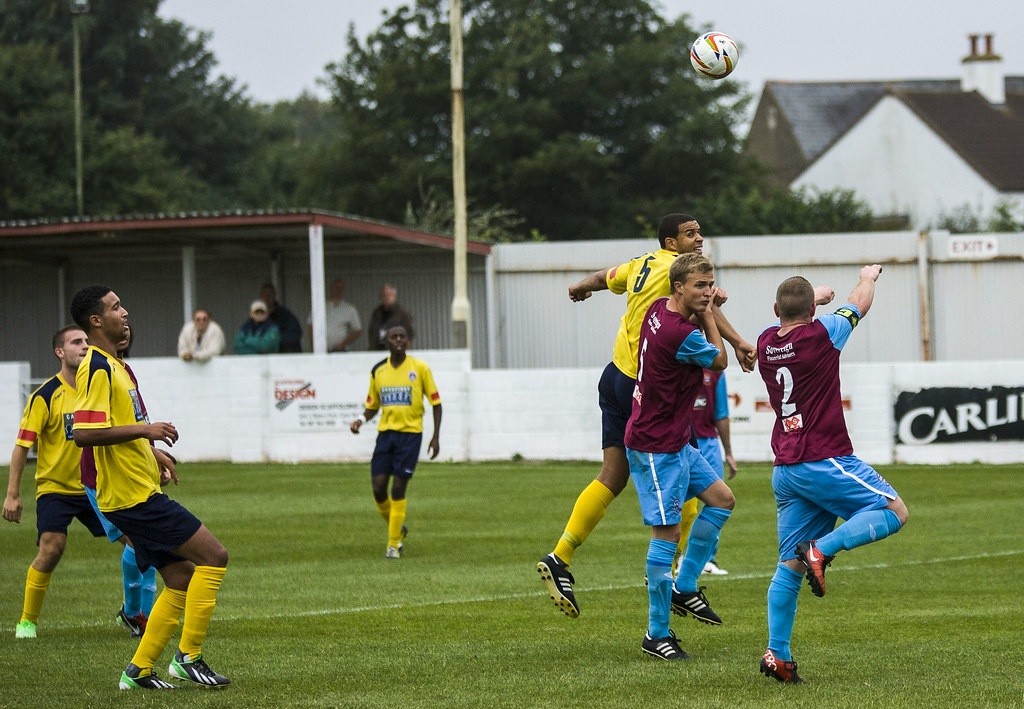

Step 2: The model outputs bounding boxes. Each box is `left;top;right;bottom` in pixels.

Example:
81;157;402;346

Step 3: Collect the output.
250;299;267;313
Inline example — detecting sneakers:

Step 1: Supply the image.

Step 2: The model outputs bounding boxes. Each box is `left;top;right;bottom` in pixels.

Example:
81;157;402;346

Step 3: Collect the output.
796;538;835;598
119;669;175;691
167;651;228;688
116;603;148;636
759;648;806;685
386;542;403;559
640;626;689;661
14;619;37;638
678;555;728;576
670;582;722;626
535;552;580;618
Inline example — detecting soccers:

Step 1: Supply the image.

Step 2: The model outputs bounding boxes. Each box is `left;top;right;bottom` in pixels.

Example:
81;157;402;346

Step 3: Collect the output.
689;31;739;80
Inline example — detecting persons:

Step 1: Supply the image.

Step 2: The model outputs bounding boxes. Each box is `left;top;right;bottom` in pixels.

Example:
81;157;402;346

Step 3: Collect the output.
758;264;910;685
70;284;230;691
233;277;415;354
625;254;736;662
2;316;178;638
537;214;758;619
177;306;227;362
350;325;442;558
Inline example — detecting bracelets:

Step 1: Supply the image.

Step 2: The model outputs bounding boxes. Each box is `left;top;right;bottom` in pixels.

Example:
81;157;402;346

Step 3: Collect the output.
358;414;366;424
